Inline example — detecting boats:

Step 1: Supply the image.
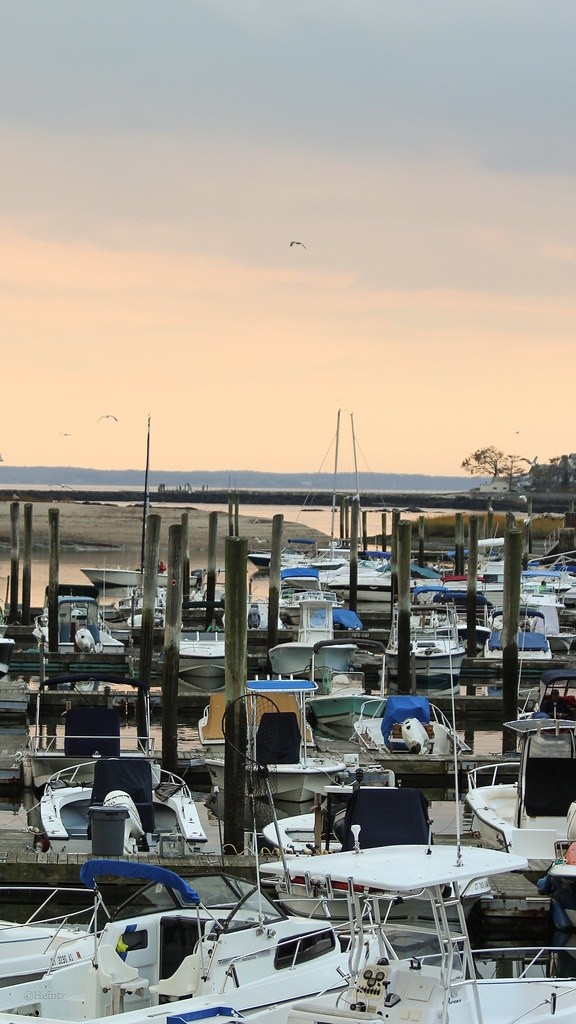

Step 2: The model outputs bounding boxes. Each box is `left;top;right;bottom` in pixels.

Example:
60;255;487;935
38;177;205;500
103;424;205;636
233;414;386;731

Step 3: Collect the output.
0;403;576;1024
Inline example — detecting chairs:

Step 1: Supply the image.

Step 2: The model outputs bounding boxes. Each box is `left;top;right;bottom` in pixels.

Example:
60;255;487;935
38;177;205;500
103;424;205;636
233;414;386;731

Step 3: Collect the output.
96;945;149;991
148;954;202;1001
